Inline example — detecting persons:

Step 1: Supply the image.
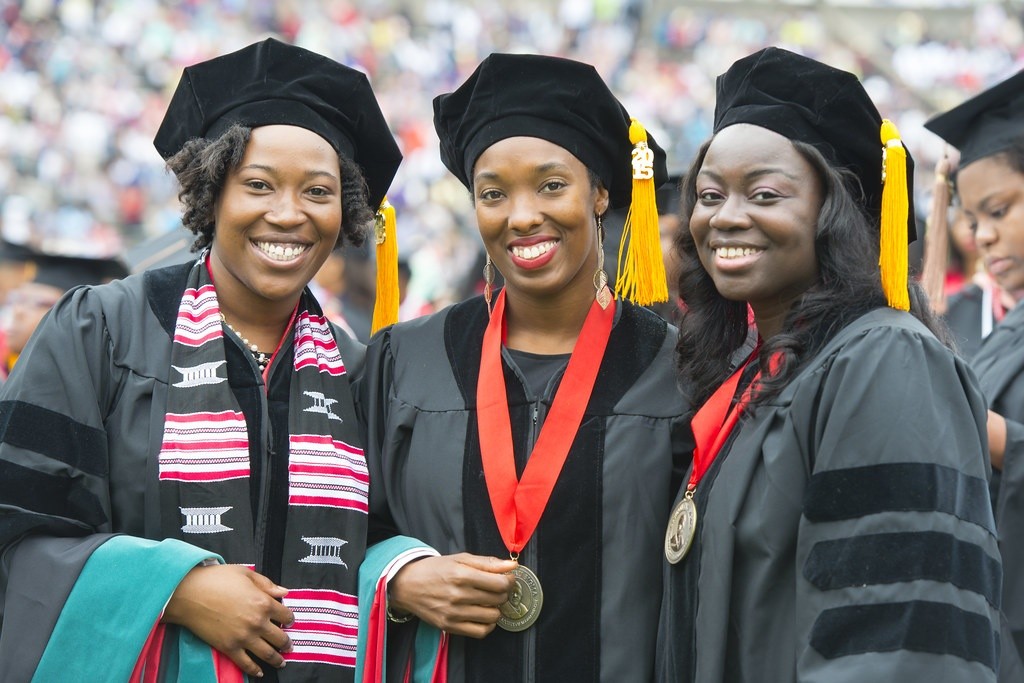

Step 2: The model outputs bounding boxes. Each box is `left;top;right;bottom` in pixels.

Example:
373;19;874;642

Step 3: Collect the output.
925;68;1024;683
0;41;403;683
651;48;1002;683
911;156;1017;361
0;238;132;381
601;171;757;372
352;52;717;683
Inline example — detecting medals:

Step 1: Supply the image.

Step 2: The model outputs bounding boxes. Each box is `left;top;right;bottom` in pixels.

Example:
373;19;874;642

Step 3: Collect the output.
488;564;543;631
664;498;697;564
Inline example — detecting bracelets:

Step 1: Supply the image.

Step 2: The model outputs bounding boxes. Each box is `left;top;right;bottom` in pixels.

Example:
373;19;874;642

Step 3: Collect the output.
385;588;415;623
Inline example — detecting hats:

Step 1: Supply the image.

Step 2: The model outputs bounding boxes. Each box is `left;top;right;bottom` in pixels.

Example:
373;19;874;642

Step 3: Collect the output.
432;53;670;307
710;47;919;313
153;37;402;339
920;68;1024;322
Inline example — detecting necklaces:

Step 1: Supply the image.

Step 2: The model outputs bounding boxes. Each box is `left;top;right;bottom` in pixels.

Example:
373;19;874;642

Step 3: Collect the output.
221;313;271;371
